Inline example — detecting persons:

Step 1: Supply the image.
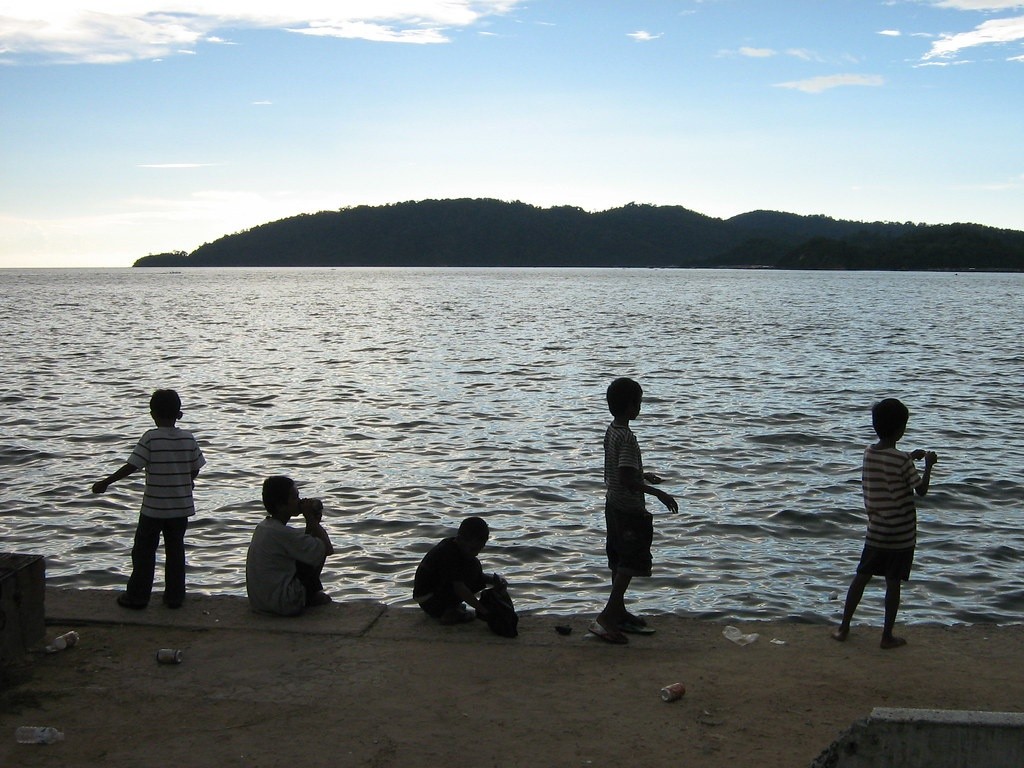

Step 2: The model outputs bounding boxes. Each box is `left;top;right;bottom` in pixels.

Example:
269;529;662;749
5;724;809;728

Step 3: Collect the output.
586;377;679;647
92;387;207;609
829;397;939;649
412;516;510;627
245;475;333;615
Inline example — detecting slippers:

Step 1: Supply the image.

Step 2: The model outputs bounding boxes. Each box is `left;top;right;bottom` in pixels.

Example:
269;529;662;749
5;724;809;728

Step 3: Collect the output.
588;621;629;644
617;613;656;634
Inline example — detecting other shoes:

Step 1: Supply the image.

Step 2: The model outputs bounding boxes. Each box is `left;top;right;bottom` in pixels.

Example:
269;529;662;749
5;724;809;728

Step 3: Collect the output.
309;593;331;607
118;594;148;610
441;608;476;626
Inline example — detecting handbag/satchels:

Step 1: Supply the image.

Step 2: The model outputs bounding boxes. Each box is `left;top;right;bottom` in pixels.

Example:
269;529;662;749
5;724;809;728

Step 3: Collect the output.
475;581;520;638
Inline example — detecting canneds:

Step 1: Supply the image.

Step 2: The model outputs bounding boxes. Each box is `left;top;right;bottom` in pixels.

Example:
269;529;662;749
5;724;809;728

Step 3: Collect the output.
156;648;184;663
661;683;685;701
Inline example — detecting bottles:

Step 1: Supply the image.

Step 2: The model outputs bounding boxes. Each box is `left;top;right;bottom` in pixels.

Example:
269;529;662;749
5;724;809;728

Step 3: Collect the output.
16;726;65;744
47;629;80;654
